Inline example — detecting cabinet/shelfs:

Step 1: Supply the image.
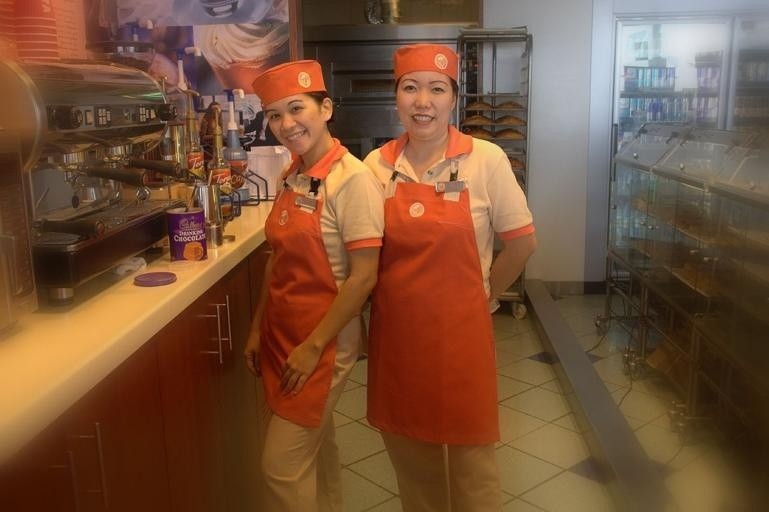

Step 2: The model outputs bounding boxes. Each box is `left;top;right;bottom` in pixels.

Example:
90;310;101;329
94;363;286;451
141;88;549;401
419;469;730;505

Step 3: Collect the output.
159;267;251;509
602;49;765;444
461;30;530;195
0;333;156;512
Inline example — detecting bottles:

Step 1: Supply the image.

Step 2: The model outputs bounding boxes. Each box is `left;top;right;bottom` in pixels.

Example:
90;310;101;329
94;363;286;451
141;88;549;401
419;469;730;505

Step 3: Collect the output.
186;88;248;223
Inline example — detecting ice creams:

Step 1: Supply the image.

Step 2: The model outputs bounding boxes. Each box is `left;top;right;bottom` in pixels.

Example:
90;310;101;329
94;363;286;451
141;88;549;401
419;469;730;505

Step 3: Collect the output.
192;2;288;93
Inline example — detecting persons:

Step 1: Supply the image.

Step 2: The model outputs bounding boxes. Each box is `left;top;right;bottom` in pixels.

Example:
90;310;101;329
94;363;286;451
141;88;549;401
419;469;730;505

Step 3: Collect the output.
362;42;538;512
243;59;386;512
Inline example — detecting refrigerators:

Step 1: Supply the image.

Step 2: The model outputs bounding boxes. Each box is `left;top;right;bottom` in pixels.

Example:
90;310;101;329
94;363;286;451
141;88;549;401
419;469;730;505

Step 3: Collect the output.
606;11;769;294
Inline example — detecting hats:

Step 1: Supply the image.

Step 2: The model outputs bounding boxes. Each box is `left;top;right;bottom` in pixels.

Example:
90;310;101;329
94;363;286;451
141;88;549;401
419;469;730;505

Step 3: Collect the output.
393;43;460;83
250;59;328;110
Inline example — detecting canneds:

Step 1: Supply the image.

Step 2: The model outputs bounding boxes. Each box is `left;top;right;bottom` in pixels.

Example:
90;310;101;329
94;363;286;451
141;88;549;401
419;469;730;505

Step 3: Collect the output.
167;206;207;262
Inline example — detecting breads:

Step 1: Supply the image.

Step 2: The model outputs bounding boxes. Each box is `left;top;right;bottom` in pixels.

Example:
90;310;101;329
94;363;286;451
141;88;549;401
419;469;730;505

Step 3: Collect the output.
632;186;738;295
460;99;526;192
